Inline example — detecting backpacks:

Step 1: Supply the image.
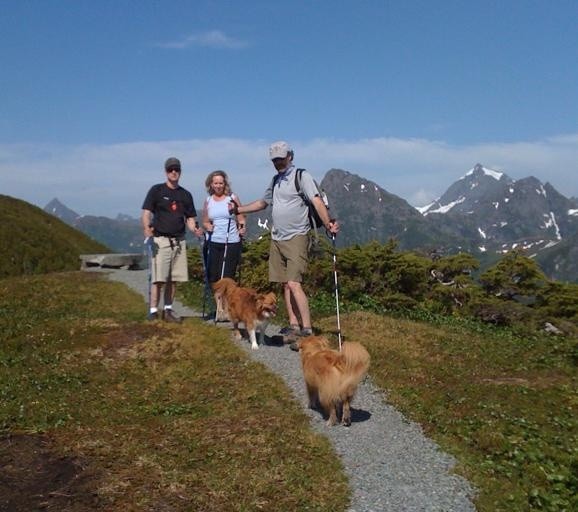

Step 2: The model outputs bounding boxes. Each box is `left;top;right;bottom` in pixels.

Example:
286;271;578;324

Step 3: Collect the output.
272;168;329;229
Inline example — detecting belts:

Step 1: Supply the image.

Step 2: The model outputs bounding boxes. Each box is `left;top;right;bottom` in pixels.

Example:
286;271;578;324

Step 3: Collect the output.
153;230;175;238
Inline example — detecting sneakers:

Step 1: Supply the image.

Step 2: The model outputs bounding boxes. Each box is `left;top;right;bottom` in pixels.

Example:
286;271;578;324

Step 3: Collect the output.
147;308;185;323
271;325;315;351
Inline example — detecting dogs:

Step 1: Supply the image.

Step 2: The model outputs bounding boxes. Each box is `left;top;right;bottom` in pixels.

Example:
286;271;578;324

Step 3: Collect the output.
296;334;372;428
211;275;281;351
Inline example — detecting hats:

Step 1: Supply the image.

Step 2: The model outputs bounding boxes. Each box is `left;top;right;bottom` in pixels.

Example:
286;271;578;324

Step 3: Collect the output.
268;141;292;161
165;157;181;170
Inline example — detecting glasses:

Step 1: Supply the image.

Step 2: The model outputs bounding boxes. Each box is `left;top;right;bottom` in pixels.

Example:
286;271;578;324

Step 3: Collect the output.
167;167;180;173
